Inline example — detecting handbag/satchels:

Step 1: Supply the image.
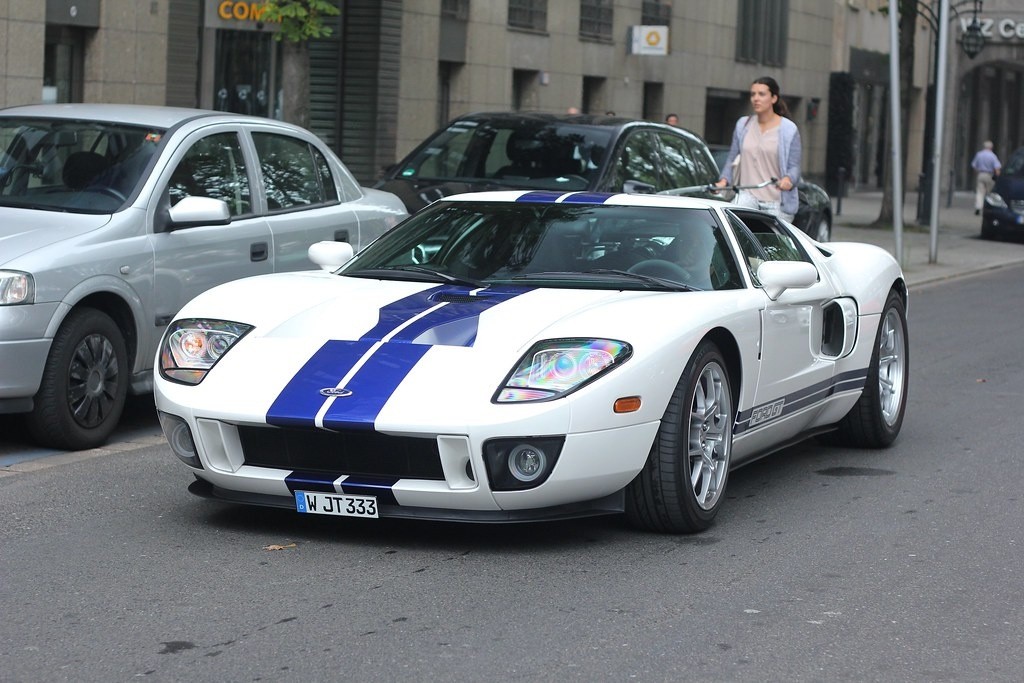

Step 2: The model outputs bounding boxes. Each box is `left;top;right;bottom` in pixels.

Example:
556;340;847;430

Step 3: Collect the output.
730;114;753;187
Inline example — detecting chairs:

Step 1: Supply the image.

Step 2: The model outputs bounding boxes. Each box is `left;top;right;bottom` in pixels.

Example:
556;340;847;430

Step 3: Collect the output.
578;144;637;193
63;150;112;192
172;156;224;211
494;133;549;184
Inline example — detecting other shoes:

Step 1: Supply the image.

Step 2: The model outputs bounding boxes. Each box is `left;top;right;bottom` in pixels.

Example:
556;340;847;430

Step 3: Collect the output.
976;210;980;215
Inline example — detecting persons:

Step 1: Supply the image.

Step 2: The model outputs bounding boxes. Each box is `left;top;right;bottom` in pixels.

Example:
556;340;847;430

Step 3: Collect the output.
664;113;680;126
567;106;580;115
605;111;617;118
711;77;803;222
972;141;1003;215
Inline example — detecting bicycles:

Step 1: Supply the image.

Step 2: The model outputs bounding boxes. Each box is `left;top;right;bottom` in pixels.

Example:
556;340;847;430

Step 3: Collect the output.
708;177;792;209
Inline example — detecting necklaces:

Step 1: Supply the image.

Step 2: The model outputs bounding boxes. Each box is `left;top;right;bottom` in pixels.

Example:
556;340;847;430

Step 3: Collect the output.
759;112;775;124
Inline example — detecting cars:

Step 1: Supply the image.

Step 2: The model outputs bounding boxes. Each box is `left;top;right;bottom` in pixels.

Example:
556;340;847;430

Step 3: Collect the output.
980;146;1024;244
373;111;737;222
0;102;410;451
710;151;833;244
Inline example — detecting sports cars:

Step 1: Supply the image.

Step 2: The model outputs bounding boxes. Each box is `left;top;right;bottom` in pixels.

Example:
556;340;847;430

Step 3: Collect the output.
153;187;910;535
